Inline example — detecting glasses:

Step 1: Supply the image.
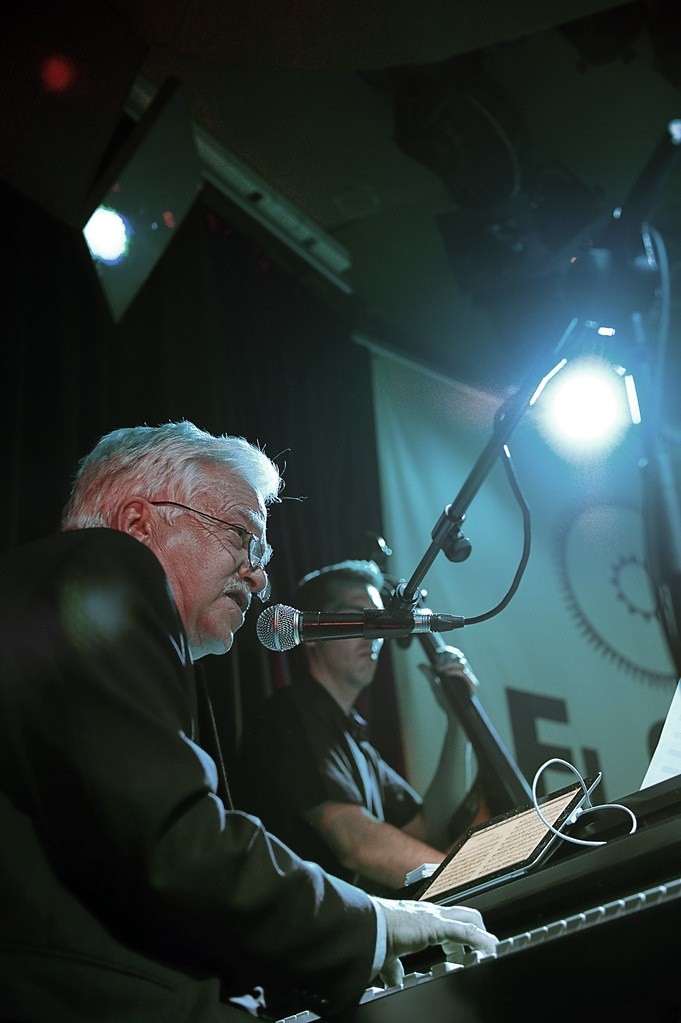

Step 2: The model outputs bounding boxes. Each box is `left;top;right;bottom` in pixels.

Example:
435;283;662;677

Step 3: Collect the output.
149;501;264;572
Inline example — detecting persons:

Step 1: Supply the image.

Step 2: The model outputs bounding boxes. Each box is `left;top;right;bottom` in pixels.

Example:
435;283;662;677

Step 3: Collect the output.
0;420;500;1023
235;561;480;900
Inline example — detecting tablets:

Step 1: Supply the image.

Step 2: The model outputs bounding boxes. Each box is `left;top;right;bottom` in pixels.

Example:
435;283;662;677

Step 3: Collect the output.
411;772;601;908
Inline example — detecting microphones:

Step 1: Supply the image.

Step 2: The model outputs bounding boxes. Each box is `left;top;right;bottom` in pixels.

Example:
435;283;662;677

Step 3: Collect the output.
256;604;463;654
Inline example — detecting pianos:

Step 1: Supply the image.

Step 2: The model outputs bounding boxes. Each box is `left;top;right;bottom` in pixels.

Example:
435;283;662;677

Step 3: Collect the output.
260;773;681;1023
